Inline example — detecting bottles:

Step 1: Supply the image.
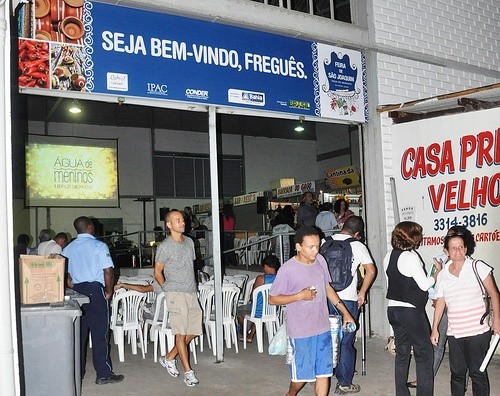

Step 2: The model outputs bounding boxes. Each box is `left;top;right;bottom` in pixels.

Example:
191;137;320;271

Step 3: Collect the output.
341;322;356;332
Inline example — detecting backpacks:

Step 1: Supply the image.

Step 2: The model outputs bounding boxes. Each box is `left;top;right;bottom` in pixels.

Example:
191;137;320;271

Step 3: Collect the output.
318;237;359;292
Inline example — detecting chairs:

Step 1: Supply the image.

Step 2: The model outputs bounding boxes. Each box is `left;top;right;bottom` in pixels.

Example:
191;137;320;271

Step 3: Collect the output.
243;285;281;354
110;267;256;365
232;236;273;266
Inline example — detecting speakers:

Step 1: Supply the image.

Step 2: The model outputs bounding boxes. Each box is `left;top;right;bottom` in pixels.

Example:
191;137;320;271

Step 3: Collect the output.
257;196;268;214
160;207;169;221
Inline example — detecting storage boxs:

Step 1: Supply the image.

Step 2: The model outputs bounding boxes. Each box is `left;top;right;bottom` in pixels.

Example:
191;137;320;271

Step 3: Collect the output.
19;255;66;306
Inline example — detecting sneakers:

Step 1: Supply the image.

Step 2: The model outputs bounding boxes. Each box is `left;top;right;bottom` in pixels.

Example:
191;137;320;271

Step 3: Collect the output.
95;374;124;385
159;356;180;378
334;384;360;395
184;370;199;387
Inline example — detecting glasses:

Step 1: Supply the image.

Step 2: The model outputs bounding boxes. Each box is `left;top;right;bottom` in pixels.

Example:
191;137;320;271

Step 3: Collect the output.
447;232;464;237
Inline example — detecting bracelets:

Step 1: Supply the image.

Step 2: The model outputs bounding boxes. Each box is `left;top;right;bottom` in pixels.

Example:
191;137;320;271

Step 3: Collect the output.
335;300;342;306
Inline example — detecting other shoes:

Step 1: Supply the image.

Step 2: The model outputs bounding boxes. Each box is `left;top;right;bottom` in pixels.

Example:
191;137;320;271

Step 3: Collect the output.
406;382;417;388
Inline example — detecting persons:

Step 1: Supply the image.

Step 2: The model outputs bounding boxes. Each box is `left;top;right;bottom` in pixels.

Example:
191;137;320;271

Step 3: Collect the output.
430;233;500;396
145;226;166;263
407;226;470;392
182;204;236;267
114;269;169;340
270;205;295;260
310;215;376;394
13;229;76;288
383;221;443;396
154;210;203;387
61;216;123;385
238;255;281;343
296;192;355;239
268;226;356;396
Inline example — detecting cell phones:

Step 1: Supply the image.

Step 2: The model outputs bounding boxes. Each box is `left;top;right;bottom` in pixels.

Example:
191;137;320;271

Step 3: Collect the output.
309;286;317;294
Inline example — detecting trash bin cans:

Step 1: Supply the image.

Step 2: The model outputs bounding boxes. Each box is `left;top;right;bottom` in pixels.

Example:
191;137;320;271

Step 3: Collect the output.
20;298;82;396
66;286;90;396
